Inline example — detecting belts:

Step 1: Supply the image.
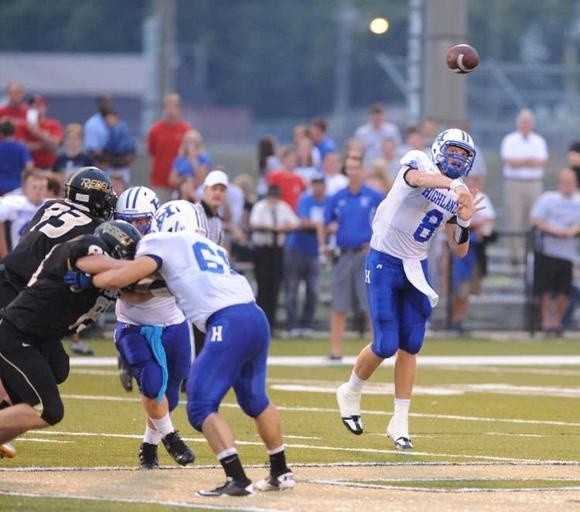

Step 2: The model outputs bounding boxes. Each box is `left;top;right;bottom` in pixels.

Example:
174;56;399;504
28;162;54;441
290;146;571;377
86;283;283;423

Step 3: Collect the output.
337;244;368;254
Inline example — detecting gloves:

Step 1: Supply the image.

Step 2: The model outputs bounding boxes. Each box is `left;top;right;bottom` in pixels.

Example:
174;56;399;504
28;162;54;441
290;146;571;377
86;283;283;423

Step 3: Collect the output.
63;271;101;292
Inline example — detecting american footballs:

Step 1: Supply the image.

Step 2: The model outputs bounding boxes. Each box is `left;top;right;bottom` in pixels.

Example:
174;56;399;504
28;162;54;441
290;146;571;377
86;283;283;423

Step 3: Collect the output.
444;43;479;74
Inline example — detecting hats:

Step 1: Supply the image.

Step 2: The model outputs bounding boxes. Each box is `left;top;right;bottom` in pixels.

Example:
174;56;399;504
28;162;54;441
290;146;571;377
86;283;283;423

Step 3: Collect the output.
203;169;229;189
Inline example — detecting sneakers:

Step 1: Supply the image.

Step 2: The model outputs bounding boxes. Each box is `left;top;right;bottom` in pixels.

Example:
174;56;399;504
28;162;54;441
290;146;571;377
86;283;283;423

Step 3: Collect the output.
302;327;314;341
196;478;256;499
385;417;414;452
324;355;343;365
136;440;160;471
289;328;300;341
71;342;93;358
161;429;198;467
252;469;296;493
0;443;18;460
117;354;135;392
336;383;366;436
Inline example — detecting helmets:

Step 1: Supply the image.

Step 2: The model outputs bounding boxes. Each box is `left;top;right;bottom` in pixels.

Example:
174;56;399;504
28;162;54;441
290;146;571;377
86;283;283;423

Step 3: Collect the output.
63;166;117;221
112;185;159;236
432;127;476;180
149;199;206;237
96;220;143;261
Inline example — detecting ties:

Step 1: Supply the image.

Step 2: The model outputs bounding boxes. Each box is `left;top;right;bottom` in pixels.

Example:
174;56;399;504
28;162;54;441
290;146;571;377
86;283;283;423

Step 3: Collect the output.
269;207;279;246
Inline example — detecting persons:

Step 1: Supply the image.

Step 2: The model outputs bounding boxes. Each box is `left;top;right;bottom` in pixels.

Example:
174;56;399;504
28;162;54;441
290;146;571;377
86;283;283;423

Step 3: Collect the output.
0;82;578;496
